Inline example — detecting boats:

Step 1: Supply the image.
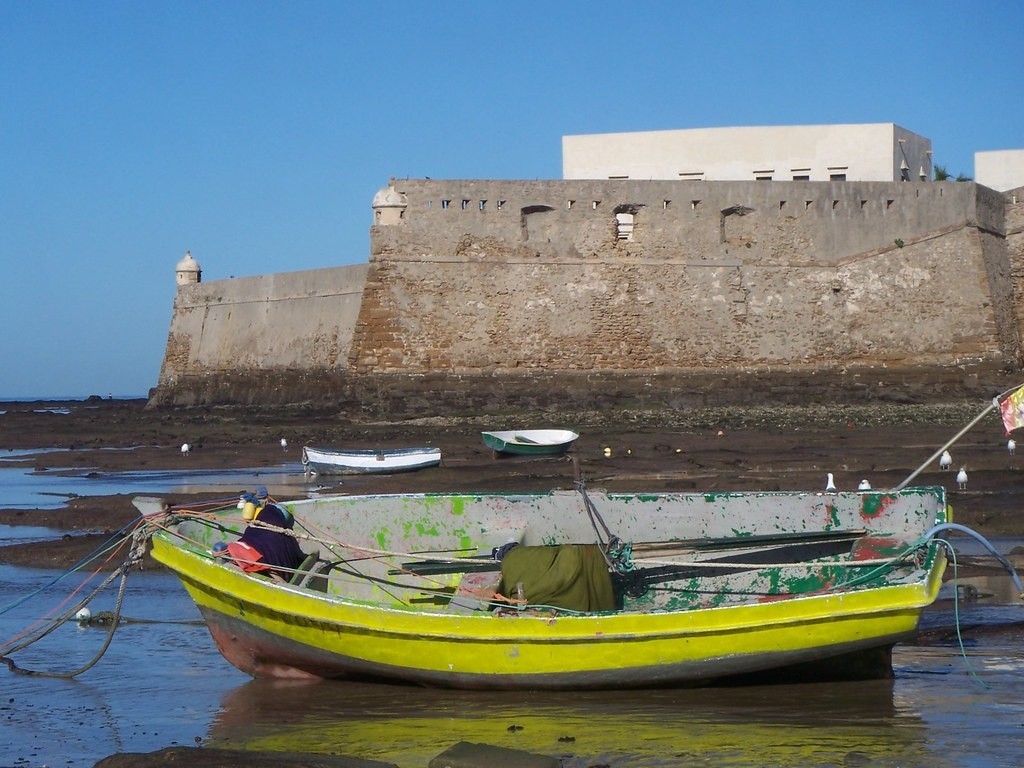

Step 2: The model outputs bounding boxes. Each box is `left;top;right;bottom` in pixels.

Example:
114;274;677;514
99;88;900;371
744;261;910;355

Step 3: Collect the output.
480;429;581;455
303;445;442;476
146;484;951;692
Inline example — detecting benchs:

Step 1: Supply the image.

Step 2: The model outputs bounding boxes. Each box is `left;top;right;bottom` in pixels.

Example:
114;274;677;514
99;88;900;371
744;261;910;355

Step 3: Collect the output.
514;435;537;443
444;530;526;611
834;531;930;587
